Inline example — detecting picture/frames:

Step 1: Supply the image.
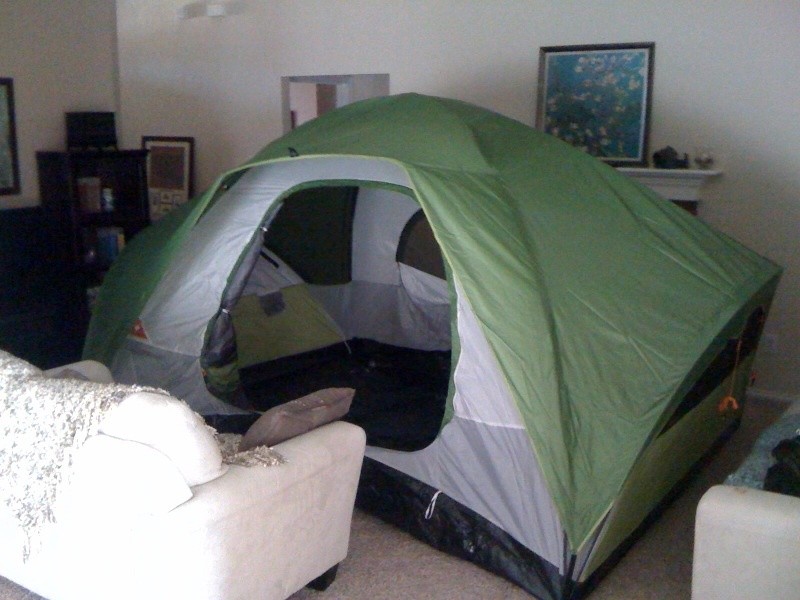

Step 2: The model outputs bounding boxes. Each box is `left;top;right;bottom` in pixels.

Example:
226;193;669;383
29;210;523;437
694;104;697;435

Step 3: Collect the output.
141;135;194;219
0;77;21;195
536;41;657;167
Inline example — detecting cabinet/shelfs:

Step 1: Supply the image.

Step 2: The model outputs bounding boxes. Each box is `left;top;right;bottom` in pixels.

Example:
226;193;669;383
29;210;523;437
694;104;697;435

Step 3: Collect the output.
36;150;149;335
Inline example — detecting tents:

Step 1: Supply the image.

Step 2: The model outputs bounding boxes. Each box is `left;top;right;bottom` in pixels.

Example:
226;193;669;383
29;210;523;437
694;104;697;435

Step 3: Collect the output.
81;92;783;600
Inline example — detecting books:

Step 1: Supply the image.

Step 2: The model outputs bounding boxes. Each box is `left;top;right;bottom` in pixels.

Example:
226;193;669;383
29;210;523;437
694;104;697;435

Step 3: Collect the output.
95;227;126;259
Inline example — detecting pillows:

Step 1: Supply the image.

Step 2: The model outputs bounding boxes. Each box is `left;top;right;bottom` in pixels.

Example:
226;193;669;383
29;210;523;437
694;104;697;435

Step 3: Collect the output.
238;386;356;451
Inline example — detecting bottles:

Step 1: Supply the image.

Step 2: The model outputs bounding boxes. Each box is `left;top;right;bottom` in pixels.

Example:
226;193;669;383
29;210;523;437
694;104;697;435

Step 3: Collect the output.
80;227;97;255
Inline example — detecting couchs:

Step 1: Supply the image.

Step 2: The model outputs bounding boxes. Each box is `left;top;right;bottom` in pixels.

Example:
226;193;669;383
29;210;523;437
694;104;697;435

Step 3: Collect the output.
691;396;800;600
0;350;367;600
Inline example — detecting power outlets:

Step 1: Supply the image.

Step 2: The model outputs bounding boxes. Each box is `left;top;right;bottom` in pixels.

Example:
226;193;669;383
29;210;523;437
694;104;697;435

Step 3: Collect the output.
762;334;778;355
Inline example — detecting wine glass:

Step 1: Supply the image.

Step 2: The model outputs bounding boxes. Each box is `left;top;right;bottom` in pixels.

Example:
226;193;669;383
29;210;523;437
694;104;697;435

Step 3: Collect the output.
102;188;114;209
